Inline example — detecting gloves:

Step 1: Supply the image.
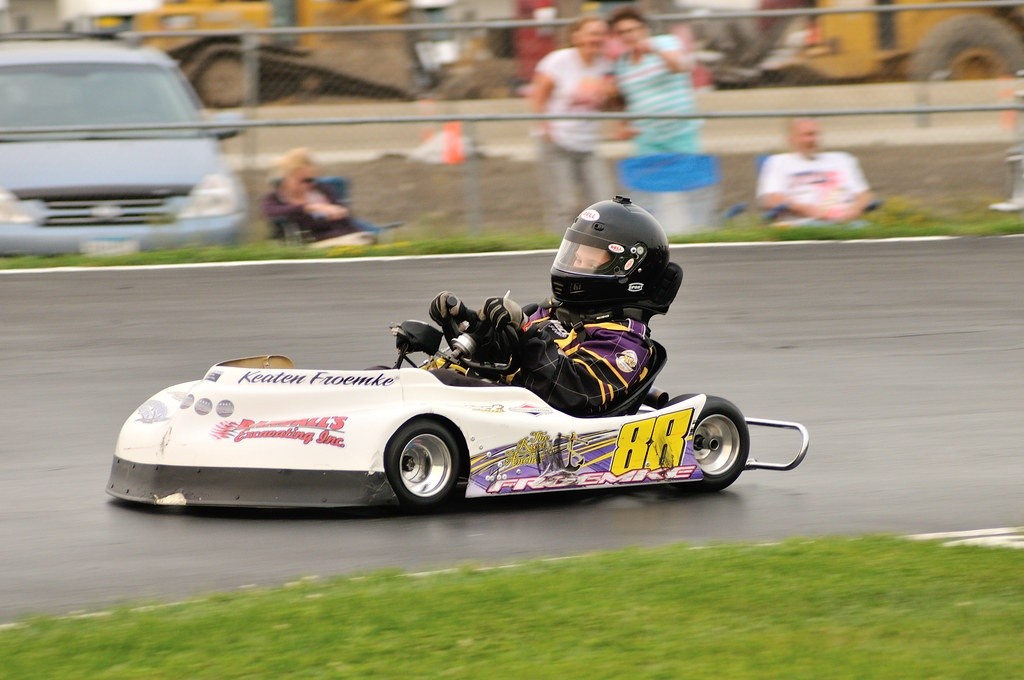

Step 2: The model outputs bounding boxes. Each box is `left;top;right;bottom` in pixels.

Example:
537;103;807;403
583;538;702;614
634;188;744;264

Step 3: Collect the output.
427;291;466;327
478;291;529;332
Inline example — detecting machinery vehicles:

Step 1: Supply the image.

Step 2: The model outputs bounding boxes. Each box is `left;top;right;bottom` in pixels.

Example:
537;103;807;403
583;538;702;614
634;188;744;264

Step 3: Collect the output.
713;2;1024;84
66;0;521;105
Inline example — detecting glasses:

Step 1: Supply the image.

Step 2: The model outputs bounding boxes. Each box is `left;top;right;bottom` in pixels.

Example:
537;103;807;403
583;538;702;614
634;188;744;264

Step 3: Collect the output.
618;25;645;34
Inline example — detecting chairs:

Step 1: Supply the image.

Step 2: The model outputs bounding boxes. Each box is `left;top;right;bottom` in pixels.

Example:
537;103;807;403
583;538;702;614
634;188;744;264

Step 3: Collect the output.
755;151;886;227
592;337;668;418
269;177;402;244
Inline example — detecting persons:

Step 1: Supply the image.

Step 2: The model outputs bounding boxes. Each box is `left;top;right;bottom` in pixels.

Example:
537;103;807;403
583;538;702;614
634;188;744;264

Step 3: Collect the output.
607;4;716;237
753;116;879;230
529;15;621;242
416;195;683;418
271;146;350;223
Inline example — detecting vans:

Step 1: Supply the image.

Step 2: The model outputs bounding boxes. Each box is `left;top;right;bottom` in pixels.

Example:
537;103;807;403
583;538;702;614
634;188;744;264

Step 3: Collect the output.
0;34;250;269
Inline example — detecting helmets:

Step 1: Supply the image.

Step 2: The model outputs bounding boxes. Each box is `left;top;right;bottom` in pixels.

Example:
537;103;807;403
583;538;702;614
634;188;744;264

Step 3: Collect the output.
549;196;682;315
